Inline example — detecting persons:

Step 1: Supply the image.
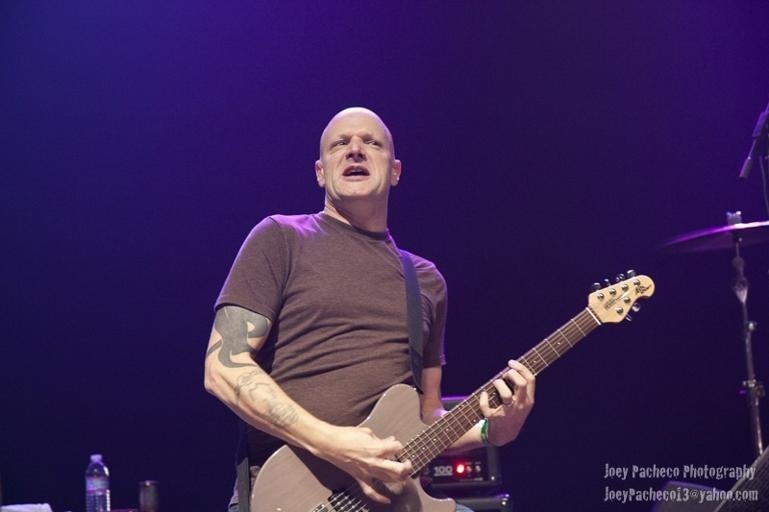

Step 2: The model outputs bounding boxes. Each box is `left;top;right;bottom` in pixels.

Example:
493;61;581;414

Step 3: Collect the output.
203;107;538;511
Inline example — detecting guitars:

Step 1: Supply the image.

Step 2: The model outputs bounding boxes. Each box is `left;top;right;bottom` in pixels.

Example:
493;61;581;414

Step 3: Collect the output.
248;270;655;511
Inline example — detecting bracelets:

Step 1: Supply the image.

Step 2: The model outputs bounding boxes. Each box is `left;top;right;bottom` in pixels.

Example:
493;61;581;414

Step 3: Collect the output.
480;418;495;448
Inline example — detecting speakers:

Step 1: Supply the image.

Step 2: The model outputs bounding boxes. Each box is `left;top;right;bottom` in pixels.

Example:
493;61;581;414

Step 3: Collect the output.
456;493;512;512
713;447;769;512
428;396;502;489
649;481;726;511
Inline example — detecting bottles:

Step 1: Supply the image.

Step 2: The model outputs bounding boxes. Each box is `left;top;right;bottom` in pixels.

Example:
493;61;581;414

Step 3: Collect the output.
85;451;110;512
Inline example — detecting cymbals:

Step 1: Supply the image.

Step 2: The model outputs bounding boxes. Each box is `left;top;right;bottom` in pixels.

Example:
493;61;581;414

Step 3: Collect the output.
660;221;769;250
